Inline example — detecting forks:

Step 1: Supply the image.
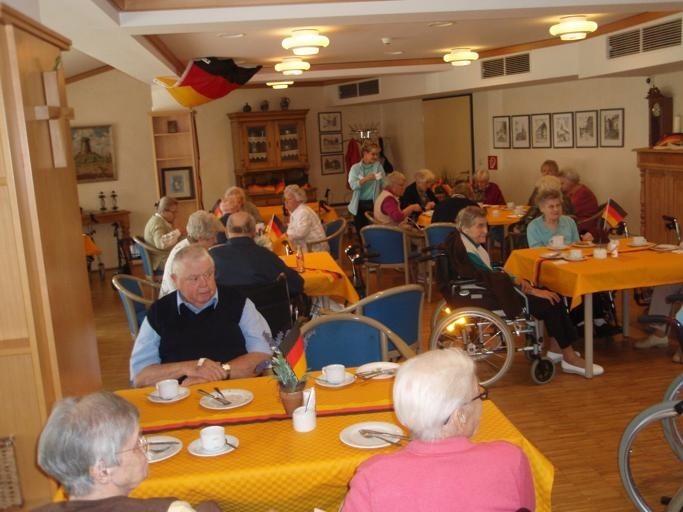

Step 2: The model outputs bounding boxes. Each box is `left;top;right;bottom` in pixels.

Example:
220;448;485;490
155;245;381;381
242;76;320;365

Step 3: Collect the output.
149;446;170;454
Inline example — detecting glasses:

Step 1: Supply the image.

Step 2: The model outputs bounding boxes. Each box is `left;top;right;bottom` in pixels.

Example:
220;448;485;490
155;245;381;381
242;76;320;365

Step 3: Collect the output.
164;209;179;214
442;379;491;425
97;435;149;463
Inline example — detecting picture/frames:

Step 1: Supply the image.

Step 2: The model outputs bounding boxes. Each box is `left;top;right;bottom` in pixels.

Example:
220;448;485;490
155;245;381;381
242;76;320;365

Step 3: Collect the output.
551;112;574;149
575;110;598;148
69;124;118;183
162;167;196;200
492;116;511;149
321;154;345;175
318;112;342;132
530;113;552;149
511;115;531;149
599;108;624;148
320;133;344;153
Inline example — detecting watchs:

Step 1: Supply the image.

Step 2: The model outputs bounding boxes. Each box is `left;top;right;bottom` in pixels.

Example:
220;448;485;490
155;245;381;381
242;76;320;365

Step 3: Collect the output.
220;359;232;381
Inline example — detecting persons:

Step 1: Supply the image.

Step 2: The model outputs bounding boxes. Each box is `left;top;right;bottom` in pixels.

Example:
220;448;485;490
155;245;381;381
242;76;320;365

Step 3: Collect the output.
373;160;599;285
347;139;386;254
525;190;623;339
128;245;275;388
447;204;605;376
339;348;536;512
635;241;683;363
23;392;222;512
144;184;329;337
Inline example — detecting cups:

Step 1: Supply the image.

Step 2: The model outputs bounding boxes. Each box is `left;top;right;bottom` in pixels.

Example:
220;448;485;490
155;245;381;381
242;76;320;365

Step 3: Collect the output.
506;201;515;208
322;364;345;384
157;379;178;400
293;407;317;432
633;236;645;246
568;250;581;258
200;425;225;450
593;249;607;259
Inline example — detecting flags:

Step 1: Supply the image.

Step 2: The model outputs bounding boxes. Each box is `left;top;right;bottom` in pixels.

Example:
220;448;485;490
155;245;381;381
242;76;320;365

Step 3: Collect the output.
602;197;627;227
281;323;308;380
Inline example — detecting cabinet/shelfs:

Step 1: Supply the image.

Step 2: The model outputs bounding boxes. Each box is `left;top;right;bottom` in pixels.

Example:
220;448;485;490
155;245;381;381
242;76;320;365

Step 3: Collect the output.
0;1;103;512
147;110;202;237
233;167;316;205
226;108;320;206
630;148;682;247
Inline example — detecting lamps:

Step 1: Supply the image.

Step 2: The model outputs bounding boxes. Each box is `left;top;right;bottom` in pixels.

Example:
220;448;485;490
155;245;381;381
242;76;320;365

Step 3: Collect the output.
281;29;330;57
442;48;480;66
266;80;294;89
99;190;107;211
549;15;598;41
111;190;120;211
274;57;310;75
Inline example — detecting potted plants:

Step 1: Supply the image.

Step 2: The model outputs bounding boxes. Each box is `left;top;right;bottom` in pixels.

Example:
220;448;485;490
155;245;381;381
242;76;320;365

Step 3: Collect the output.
264;331;310;418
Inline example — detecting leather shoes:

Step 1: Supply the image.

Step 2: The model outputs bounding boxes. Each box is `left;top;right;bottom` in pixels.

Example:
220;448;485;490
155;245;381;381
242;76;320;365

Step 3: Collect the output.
673;348;681;363
545;351;580;366
633;335;669;349
561;356;606;378
593;322;624;337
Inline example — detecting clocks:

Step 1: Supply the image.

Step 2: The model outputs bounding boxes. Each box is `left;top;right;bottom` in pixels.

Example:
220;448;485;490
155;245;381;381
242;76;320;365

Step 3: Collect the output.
644;83;673;149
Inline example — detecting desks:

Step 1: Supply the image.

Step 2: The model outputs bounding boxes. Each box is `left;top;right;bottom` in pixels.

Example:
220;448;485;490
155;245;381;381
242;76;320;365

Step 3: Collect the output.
81;208;132;285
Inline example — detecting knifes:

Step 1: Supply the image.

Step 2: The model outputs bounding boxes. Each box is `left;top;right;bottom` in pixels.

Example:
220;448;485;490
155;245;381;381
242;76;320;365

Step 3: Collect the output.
196;388;232;406
147;442;180;445
362;429;412;440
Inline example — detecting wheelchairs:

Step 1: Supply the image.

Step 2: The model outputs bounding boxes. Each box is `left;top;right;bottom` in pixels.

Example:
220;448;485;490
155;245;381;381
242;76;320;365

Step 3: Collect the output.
212;272;298;342
617;293;683;510
412;248;556;384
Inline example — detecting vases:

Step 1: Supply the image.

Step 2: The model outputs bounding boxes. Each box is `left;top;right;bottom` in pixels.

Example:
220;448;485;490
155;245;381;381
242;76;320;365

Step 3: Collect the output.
243;102;251;112
280;97;289;111
260;100;269;111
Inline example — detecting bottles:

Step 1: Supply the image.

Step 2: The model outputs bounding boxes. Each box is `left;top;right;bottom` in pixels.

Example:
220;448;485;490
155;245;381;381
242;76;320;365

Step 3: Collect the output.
248;139;268;162
295;245;304;271
281;136;299;160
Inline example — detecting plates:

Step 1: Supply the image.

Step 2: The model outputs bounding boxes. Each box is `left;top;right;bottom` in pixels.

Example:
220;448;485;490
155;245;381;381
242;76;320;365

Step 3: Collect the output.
539;253;565;259
357;361;400;380
649;244;678;251
315;371;357;388
147;387;191;404
506;214;523;218
625;243;650;247
548;245;571;251
562;254;589;261
572;242;595;248
200;389;254;409
188;435;238;456
549;236;564;247
338;421;405;450
144;436;183;463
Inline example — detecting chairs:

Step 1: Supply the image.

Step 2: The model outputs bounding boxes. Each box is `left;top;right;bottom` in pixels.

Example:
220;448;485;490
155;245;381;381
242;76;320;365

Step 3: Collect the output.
359;225;414;298
112;274;160;342
364;210;382;225
132;235;170;303
336;284;426;362
306;217;347;269
423;223;460;303
266;313;416;376
